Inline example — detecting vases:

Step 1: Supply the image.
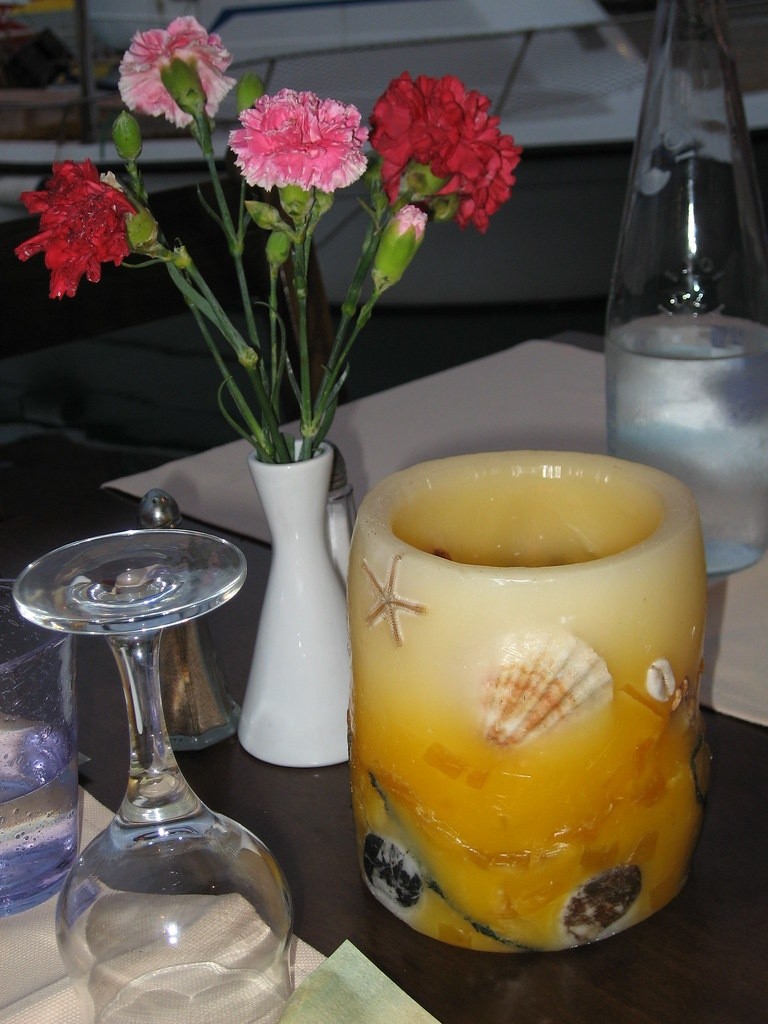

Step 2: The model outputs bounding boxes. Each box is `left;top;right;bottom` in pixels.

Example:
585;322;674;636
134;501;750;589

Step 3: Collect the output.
237;439;355;769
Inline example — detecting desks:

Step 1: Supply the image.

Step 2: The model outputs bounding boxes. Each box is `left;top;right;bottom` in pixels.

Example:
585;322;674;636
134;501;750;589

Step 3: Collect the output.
1;136;768;1024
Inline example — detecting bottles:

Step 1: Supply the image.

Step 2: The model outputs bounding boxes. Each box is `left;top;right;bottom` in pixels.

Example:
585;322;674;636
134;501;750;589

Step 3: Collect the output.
139;488;239;753
603;0;768;577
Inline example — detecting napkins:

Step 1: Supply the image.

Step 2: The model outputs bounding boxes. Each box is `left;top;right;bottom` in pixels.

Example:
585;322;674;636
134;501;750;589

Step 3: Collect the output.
97;338;768;728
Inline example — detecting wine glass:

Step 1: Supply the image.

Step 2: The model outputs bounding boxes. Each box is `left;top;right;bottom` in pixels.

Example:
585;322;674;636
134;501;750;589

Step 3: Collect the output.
13;527;295;1024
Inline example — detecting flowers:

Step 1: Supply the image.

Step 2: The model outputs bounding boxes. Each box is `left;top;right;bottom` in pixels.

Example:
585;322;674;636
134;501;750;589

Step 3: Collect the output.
17;13;522;464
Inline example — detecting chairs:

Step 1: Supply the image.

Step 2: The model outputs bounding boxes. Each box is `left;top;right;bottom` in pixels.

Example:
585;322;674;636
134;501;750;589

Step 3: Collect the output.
173;2;717;151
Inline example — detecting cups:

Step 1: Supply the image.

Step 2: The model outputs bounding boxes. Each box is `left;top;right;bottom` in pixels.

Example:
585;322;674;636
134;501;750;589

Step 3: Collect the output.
347;450;709;951
0;577;80;917
